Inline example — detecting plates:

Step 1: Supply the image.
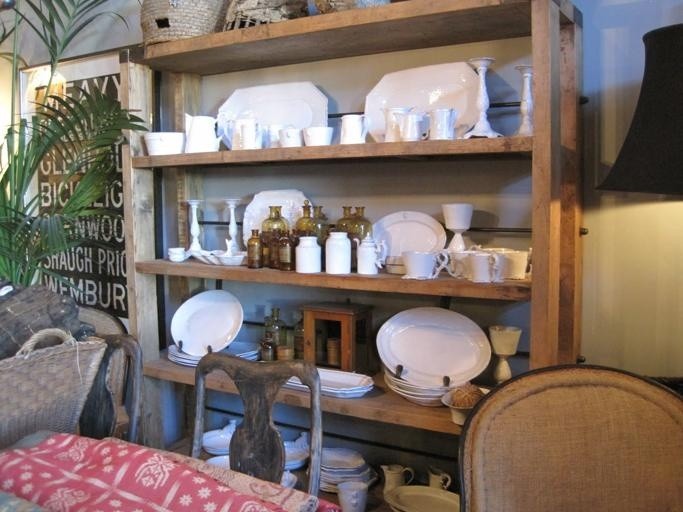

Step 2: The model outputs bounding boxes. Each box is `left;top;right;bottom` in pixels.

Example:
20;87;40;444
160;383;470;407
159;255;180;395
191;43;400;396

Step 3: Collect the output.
306;448;378;492
243;189;314;247
217;81;329;150
171;290;243;357
372;211;447;265
385;486;459;512
377;307;492;387
383;367;471;407
168;341;261;367
364;62;480;142
281;368;374;399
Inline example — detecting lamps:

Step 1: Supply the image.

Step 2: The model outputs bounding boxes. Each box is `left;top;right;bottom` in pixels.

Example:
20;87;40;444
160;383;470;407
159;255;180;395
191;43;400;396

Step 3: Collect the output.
593;22;682;196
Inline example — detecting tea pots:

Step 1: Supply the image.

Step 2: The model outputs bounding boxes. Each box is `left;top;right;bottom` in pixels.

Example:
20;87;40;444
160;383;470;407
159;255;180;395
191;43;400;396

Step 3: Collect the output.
425;466;451;490
184;112;227;153
222;108;263;150
380;465;414;503
394;112;428;141
353;232;388;274
380;104;416;142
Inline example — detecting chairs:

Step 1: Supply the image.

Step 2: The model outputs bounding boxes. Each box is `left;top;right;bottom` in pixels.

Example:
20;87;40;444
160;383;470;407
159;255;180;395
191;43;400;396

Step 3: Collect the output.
191;352;322;498
457;363;683;512
81;331;143;445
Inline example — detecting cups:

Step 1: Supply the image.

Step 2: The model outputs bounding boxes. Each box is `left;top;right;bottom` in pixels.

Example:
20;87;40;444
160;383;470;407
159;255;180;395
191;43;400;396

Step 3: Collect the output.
401;251;448;280
168;247;185;262
340;115;371;144
338;482;368;512
304;126;334;146
429;108;458;140
279;129;305;147
437;248;528;282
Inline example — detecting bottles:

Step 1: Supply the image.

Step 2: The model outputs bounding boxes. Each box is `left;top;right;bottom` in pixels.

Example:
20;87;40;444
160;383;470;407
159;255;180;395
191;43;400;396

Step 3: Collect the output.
307;206;327;236
297;201;312;236
290;229;300;246
265;308;286;345
261;332;274;361
294;312;305;361
348;207;372;272
335;206;354;233
262;206;288;267
262;239;271;267
279;230;294;271
247;230;262;269
270;229;281;269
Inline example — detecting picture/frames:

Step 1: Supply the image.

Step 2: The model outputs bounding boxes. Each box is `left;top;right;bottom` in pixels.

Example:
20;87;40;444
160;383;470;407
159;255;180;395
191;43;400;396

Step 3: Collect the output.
18;41;141;336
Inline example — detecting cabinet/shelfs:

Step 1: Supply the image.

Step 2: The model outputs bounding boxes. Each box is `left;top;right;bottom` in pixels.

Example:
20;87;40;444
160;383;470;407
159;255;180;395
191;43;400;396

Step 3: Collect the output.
124;0;582;483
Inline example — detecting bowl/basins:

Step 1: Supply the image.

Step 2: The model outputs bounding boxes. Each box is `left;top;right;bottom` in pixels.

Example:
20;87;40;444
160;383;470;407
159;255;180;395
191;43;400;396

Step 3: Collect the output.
192;250;244;266
203;430;232;456
144;132;186;155
282;441;310;470
